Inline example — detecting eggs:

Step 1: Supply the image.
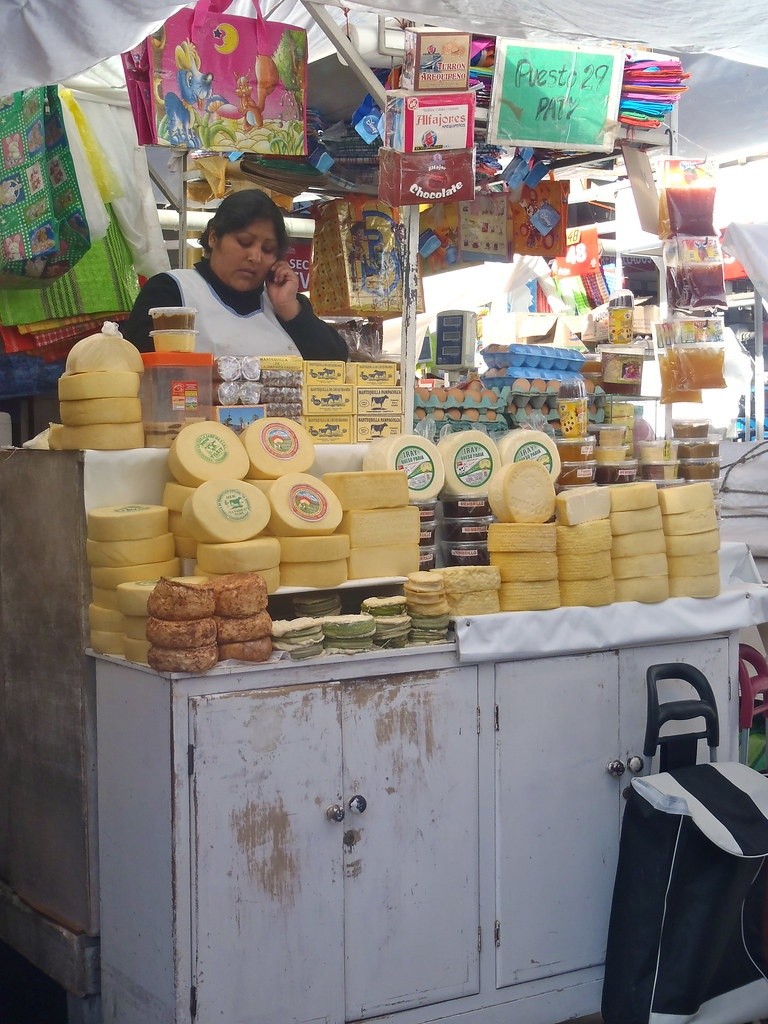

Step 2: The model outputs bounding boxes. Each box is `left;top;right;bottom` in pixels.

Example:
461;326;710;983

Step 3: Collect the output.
415;344;597;432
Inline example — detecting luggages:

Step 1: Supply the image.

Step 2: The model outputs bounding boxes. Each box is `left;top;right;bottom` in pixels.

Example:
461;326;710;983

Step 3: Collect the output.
601;663;768;1024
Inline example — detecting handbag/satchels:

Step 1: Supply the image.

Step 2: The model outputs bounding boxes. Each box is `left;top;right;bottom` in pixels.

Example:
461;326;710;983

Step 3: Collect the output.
0;85;92;279
308;194;426;319
121;0;308;158
417;167;569;279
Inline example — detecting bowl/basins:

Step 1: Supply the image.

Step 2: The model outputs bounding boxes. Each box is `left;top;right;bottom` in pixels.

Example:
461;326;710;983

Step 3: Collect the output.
549;418;724;533
148;329;200;352
579;354;603;389
407;495;496;571
148;306;198;331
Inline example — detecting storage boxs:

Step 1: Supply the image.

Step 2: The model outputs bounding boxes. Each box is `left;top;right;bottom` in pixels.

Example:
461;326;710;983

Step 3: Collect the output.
375;26;476;208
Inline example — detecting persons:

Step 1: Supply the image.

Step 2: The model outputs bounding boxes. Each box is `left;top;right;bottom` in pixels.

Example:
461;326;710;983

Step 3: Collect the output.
123;188;350;362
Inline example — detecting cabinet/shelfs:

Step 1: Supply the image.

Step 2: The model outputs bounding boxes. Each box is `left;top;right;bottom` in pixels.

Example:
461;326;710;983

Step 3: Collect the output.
88;597;743;1024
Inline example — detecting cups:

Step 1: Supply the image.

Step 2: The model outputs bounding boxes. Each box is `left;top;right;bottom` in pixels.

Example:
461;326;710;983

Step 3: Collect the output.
556;378;588;437
607;288;635;344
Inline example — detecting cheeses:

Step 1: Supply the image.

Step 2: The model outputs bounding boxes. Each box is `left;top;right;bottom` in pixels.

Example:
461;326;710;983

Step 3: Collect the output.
47;416;722;675
48;332;146;449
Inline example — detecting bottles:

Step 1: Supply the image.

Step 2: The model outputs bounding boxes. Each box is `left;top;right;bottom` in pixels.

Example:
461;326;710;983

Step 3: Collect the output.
630;406;656;460
